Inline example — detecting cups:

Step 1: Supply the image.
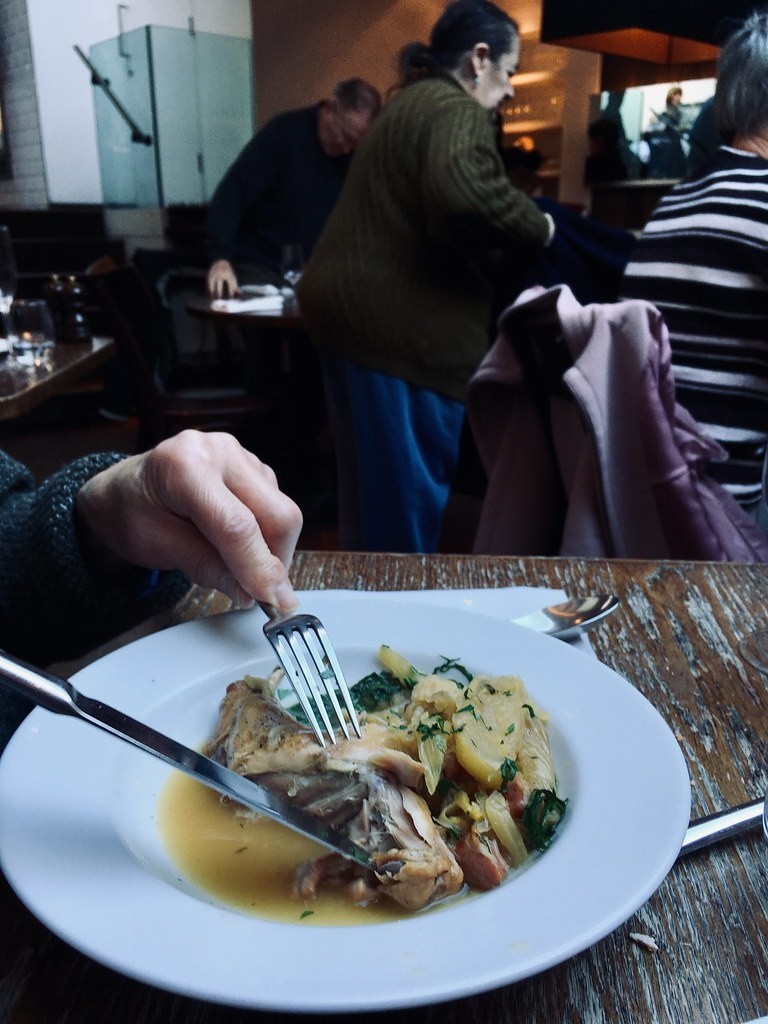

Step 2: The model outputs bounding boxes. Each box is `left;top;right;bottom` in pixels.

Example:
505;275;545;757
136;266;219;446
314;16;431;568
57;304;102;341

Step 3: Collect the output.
0;220;57;365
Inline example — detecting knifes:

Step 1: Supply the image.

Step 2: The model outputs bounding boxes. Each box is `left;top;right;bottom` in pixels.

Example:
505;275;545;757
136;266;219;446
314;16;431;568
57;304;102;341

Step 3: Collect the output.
0;652;374;871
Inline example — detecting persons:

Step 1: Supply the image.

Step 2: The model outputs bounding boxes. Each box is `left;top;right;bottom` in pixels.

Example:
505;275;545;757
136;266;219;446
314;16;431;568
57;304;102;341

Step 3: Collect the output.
617;12;768;536
650;87;685;128
295;0;555;553
0;429;304;658
198;77;382;300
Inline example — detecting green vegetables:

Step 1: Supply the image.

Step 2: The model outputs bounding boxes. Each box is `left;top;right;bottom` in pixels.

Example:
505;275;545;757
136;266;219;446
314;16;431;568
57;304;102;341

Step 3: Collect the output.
276;654;570;868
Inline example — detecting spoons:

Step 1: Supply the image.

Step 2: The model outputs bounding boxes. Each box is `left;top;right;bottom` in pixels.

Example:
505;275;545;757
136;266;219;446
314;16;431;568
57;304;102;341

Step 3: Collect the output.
510;595;620;641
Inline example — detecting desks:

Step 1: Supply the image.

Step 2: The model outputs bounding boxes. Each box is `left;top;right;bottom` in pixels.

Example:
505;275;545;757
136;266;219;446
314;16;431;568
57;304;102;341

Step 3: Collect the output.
2;554;768;1024
185;287;316;424
0;334;110;425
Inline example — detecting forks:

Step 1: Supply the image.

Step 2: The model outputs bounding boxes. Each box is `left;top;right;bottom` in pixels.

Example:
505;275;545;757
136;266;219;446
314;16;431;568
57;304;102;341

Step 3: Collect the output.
257;600;362;748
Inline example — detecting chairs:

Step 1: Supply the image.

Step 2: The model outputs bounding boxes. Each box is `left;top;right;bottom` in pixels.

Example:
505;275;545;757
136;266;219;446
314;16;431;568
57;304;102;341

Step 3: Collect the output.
447;280;768;562
83;274;319;530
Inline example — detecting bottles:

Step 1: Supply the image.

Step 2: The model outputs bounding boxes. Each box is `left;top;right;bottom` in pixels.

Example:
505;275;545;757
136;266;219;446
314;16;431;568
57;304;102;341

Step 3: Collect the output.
64;275;91;343
43;274;68;326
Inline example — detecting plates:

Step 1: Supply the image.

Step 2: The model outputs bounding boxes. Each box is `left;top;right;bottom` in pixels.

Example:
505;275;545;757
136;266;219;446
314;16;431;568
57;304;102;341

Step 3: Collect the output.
0;590;692;1014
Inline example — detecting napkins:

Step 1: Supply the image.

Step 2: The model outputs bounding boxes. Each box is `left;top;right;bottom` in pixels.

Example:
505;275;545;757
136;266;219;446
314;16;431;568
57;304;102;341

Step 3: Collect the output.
273;586;595;657
